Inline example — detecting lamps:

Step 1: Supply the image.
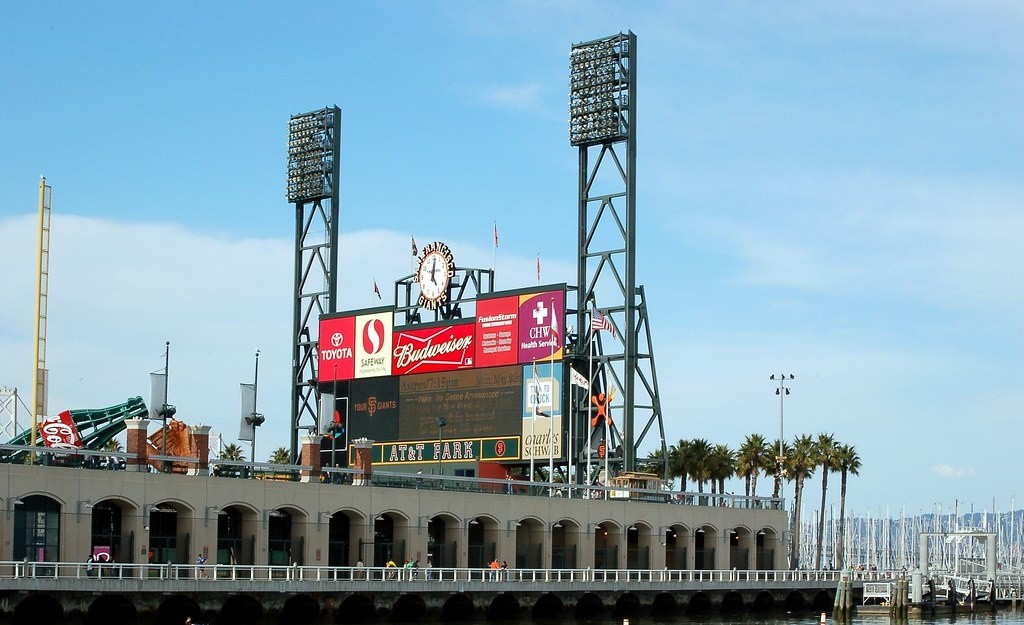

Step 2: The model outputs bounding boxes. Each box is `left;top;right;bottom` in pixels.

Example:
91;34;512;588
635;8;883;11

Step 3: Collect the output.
754;528;766;543
77;498;94;523
143;503;159;525
507;519;521;538
587;522;601;537
369;512;385;533
548;521;562;538
318;510;333;530
624;523;637;540
693;526;705;542
782;529;795;544
464;518;479;536
205;505;226;527
263;508;281;529
418;515;432;534
6;496;24;521
724;527;736;543
659;526;671;542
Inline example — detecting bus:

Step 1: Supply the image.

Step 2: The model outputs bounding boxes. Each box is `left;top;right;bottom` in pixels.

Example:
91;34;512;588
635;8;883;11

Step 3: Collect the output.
609;472;661;500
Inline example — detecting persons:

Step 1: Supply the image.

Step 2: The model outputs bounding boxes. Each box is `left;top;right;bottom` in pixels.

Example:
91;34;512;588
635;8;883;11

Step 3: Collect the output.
487;558;509;582
801;564;908;580
85;554;116;579
415;468;424;490
195;554;208;580
356;558;432;581
507;475;514;495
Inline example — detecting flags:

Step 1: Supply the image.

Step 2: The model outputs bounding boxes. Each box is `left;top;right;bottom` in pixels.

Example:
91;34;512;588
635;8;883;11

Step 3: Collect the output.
374;280;381;299
537;257;541;280
533;365;540;398
495;223;498;247
412;236;418;256
550;302;559;345
592;307;616;340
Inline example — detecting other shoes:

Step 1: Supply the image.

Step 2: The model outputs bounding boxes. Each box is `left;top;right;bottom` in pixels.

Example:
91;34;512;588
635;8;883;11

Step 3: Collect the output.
207;576;209;580
198;578;202;580
389;578;392;581
416;486;418;489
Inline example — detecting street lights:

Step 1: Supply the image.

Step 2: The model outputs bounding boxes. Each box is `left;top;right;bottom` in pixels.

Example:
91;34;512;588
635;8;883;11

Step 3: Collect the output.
771;374;795;509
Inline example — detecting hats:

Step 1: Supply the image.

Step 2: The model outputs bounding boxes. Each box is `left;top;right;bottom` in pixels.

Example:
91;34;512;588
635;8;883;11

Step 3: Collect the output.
416;560;419;562
406;560;409;562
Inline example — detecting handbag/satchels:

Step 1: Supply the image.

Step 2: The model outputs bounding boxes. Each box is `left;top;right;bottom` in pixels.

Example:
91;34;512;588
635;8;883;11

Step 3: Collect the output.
389;564;394;567
416;476;423;480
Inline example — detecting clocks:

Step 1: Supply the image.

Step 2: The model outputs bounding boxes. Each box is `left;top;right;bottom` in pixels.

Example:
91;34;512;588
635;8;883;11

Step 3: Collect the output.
419;252;448;300
